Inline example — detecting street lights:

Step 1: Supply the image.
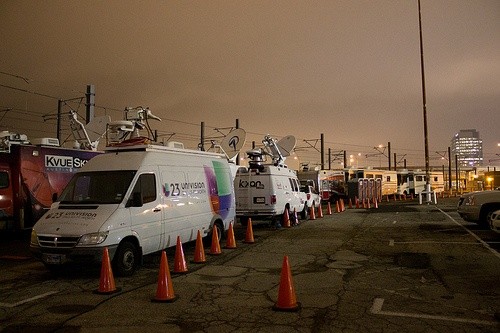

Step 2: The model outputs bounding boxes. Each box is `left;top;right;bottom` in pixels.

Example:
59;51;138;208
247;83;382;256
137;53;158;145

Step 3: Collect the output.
281;208;291;228
292;208;300;226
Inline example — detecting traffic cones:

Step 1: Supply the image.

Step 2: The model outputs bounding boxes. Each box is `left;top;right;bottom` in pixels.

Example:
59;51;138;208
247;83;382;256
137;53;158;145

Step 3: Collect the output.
94;247;123;296
241;218;257;245
223;220;240;250
325;202;334;217
203;225;224;257
170;235;192;276
151;251;181;306
308;204;319;221
187;229;208;265
273;256;302;314
316;204;324;218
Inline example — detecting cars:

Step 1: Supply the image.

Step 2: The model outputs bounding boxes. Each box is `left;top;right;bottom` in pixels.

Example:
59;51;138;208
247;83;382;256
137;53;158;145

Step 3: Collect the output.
457;187;500;231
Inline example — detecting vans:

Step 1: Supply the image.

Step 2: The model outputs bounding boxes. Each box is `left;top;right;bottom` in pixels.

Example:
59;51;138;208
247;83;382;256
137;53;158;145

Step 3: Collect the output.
234;134;308;226
29;106;237;277
296;162;447;213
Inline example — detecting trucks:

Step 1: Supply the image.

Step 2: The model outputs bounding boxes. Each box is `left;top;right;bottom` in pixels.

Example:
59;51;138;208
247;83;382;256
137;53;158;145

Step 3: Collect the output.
0;109;105;249
